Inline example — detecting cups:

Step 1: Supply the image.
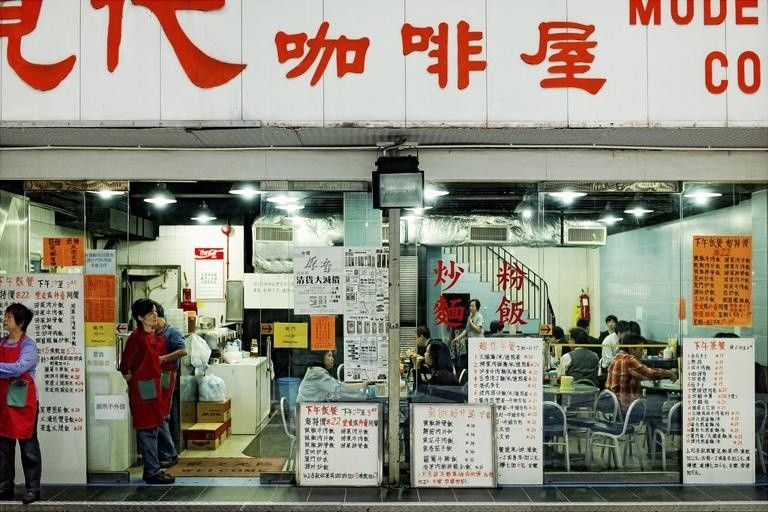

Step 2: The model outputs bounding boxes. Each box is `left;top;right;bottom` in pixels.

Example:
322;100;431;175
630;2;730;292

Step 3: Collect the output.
647;350;673;359
243;352;250;358
209;356;223;365
367;381;413;399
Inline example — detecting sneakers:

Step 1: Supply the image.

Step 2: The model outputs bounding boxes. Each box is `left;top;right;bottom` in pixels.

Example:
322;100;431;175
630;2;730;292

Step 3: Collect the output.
143;471;177;485
21;489;40;505
0;484;15;498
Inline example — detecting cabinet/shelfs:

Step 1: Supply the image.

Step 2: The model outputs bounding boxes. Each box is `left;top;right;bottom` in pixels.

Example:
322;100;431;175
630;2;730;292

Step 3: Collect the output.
204;356;274;436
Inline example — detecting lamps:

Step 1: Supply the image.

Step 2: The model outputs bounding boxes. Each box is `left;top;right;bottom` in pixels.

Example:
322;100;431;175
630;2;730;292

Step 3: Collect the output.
370;143;425;212
139;183;659;227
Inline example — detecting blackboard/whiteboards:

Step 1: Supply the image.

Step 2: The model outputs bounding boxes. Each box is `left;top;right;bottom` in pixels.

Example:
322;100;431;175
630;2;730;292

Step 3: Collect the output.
682;338;755;486
0;274;88;486
410;338;544;489
299;401;383;487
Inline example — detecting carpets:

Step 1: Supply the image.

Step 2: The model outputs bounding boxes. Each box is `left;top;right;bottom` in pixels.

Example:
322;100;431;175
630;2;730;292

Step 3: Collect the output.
166;456;287;478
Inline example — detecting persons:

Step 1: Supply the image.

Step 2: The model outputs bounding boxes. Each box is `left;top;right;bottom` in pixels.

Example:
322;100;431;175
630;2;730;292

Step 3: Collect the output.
120;298;175;484
0;303;42;504
289;350;370;437
401;298;504;470
147;299;188;468
545;314;768;459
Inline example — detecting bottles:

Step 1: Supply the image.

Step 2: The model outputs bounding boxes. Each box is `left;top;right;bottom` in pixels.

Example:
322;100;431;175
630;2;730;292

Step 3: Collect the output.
399;346;406;371
251;339;258;359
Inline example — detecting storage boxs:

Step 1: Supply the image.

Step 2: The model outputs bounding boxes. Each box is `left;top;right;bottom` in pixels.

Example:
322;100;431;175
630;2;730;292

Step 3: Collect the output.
186;398;233;450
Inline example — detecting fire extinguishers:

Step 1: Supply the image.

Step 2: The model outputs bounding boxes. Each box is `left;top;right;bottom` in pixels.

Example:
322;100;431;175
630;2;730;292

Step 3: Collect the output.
581;289;589;321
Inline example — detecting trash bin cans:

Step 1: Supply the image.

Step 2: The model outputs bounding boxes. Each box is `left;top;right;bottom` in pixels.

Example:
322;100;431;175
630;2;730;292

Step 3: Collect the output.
277;377;302;415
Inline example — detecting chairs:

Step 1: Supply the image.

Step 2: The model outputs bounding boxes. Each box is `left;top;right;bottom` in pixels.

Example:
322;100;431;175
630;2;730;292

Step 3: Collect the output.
278;347;468;471
543;337;768;474
